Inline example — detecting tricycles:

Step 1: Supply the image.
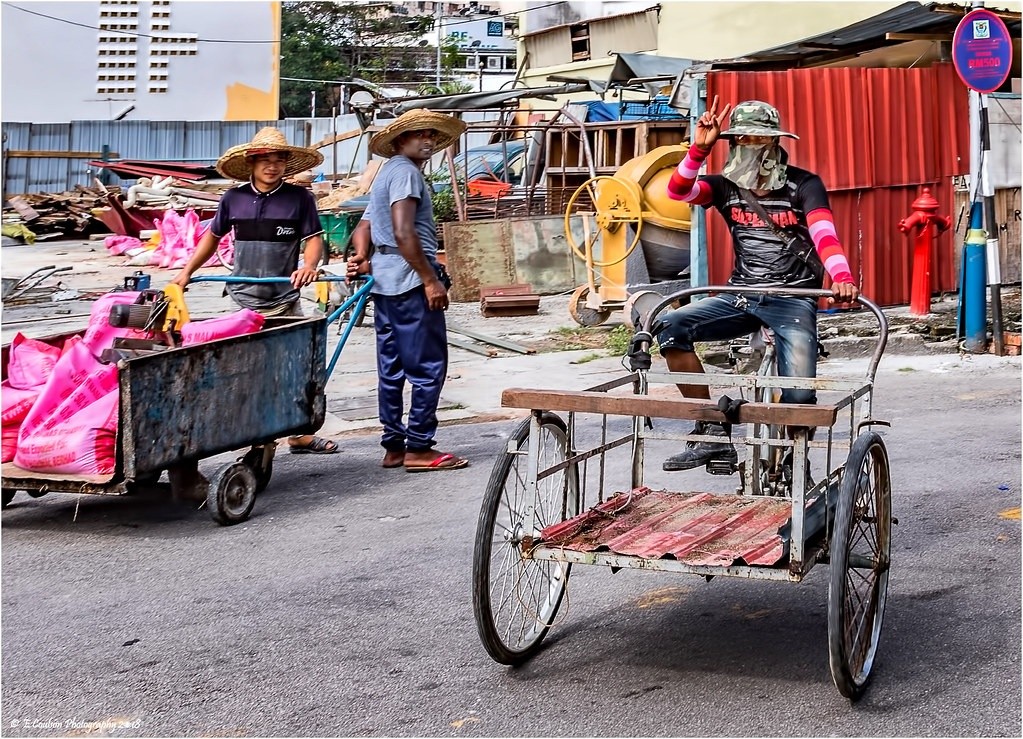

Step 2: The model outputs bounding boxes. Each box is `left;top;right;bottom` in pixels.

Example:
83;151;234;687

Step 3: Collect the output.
472;285;894;699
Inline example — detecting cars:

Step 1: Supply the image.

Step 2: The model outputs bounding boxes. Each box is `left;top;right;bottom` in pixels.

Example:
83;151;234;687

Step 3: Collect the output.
337;138;531;203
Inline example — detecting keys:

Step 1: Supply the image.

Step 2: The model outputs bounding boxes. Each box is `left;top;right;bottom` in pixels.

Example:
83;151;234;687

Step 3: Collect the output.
734;297;748;311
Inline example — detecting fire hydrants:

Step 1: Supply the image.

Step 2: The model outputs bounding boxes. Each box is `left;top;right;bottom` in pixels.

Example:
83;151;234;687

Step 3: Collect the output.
898;187;952;315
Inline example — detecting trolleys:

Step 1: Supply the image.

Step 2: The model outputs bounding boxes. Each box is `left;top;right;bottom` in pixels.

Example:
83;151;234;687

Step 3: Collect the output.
1;274;375;526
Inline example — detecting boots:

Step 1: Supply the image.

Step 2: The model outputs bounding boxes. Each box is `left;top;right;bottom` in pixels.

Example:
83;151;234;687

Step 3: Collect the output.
662;420;739;472
783;451;821;502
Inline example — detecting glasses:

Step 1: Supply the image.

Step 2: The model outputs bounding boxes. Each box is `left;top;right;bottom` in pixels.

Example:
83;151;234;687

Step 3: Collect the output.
734;135;773;145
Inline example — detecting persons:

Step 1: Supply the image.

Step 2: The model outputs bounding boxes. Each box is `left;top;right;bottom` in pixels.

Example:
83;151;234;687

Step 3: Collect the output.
170;127;338;454
657;95;861;498
346;109;469;473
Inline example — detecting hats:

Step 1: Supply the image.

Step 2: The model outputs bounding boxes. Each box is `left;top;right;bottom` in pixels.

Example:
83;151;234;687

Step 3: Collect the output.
216;126;325;182
720;101;800;140
368;108;466;160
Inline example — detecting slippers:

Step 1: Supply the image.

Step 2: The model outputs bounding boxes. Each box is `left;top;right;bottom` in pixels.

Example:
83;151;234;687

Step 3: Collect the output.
406;452;469;471
289;436;338;454
383;446;407;468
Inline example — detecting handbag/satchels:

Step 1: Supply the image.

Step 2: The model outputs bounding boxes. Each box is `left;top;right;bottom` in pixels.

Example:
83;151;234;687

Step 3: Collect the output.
381;243;451;289
